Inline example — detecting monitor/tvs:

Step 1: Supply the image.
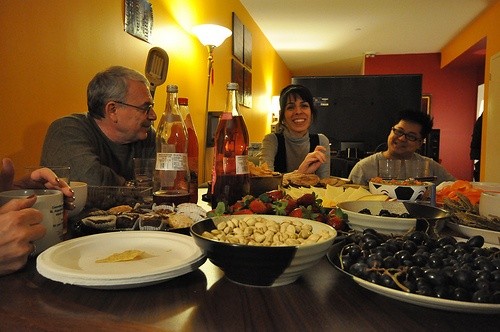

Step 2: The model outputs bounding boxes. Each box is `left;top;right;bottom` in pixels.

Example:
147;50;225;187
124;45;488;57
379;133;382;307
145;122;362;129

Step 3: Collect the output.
290;74;423;157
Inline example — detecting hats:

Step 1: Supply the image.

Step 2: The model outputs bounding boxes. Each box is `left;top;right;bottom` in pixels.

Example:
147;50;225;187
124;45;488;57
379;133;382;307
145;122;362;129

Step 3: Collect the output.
279;84;313;106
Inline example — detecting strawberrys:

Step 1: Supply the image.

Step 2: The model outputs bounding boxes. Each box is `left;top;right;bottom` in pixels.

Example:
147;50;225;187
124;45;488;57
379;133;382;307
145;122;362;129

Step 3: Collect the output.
206;188;348;230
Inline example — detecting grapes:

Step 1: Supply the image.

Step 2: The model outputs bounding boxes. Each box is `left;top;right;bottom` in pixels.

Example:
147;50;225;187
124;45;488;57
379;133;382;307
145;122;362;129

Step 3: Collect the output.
336;228;500;306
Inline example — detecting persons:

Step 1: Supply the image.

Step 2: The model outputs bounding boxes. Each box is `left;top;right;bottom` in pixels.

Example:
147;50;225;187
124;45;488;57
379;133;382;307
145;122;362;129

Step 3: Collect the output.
0;158;76;275
349;109;448;186
40;65;157;187
259;84;330;178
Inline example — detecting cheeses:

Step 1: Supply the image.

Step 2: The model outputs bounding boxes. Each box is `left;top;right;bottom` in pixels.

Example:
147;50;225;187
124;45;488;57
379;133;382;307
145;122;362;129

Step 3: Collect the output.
281;183;397;208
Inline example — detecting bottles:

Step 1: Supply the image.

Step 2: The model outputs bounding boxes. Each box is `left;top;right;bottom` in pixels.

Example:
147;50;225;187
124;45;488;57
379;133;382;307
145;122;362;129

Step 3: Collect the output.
178;98;199;205
211;82;251;215
152;84;191;204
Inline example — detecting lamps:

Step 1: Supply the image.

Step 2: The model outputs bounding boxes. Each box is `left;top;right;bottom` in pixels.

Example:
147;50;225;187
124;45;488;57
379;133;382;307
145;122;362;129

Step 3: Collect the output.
190;23;234;179
271;95;281;122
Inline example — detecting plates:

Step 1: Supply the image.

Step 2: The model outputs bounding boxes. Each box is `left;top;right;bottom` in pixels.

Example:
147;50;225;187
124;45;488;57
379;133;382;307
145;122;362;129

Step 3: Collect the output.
36;231;207;290
446;221;500;246
73;215;191;236
325;236;500;315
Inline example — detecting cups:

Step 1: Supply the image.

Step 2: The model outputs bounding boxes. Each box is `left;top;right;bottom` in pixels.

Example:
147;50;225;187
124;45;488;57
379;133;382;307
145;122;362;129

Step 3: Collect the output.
377;158;429;178
0;187;64;255
22;165;71;234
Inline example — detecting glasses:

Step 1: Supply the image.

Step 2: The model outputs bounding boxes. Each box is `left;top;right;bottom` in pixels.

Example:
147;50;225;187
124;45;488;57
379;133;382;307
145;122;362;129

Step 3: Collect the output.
391;126;424;143
106;99;155;116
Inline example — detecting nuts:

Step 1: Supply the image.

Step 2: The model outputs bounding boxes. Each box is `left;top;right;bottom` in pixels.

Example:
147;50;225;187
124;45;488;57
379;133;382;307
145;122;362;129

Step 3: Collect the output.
200;216;328;246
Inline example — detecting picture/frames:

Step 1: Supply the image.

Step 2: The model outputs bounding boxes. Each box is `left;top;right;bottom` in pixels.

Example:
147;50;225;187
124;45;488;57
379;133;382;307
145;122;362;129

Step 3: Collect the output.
231;11;254;108
422;94;432;116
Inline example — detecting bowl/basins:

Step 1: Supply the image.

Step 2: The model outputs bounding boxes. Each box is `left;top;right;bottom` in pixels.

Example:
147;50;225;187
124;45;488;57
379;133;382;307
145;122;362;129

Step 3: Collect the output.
68;181;88;219
189;214;338;288
336;201;450;237
479;192;500;219
368;178;434;202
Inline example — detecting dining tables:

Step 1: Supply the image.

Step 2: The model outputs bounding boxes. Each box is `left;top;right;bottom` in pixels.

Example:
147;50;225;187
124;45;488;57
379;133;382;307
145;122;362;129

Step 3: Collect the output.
0;187;500;332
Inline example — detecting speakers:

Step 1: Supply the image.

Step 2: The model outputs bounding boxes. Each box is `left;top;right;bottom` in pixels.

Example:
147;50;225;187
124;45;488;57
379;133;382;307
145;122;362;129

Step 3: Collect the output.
271;125;284;133
414;129;440;163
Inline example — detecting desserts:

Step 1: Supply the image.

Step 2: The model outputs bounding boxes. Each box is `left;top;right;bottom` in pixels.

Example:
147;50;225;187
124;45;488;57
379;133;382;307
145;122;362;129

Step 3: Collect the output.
81;203;208;231
